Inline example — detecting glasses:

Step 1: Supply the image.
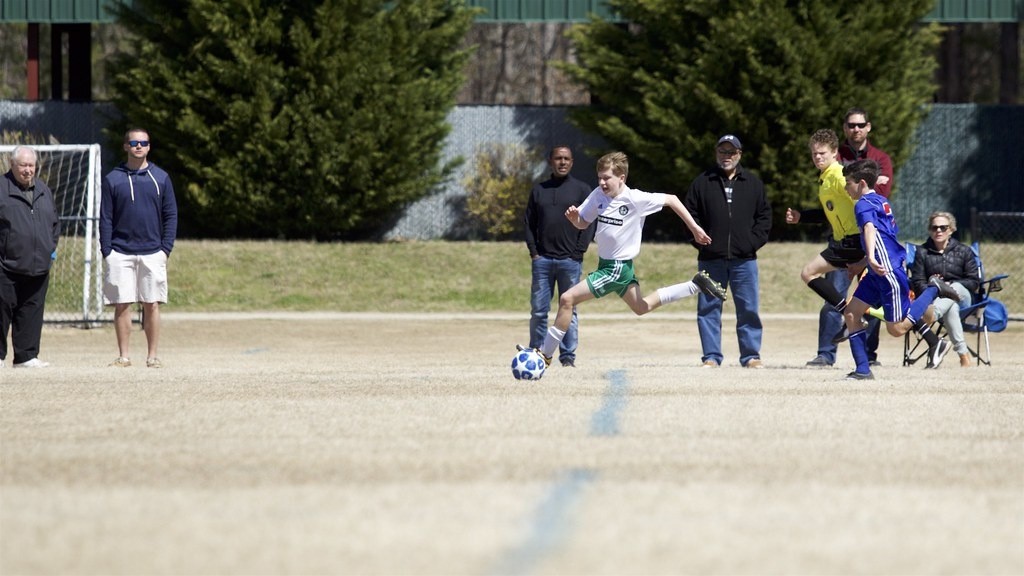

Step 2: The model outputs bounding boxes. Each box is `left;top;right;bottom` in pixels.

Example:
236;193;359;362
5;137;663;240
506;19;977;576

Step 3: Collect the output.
932;225;949;232
128;141;149;147
719;149;737;156
847;123;867;128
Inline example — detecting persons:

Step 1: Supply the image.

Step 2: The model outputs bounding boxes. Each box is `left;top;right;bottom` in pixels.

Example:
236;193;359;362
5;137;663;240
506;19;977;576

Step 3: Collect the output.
787;129;952;370
100;129;177;367
517;154;727;368
841;159;958;381
911;211;979;367
0;146;60;368
808;107;892;366
686;135;772;369
524;144;596;368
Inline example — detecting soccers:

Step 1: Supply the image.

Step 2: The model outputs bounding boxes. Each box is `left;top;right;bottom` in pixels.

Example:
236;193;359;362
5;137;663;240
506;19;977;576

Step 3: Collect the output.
510;348;546;382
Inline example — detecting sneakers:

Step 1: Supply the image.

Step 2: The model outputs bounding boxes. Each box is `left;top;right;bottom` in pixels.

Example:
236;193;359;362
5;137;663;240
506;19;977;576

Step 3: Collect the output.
832;316;869;343
806;355;832;368
692;270;727;301
516;343;552;368
928;273;961;302
925;337;951;369
868;358;881;368
845;371;875;381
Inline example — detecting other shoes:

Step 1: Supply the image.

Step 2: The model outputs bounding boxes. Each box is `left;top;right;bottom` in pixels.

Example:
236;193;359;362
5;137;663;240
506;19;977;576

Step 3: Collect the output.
701;358;717;371
147;358;162;369
110;356;131;370
561;357;576;367
960;358;970;368
746;358;764;369
14;358;50;368
923;305;935;325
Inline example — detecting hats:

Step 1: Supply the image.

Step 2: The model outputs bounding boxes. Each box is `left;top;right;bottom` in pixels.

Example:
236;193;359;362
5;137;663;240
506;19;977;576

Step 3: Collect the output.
717;135;741;150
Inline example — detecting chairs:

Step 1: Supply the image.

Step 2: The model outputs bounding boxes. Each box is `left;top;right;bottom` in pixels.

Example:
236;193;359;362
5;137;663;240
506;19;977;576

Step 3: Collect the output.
903;241;1009;368
